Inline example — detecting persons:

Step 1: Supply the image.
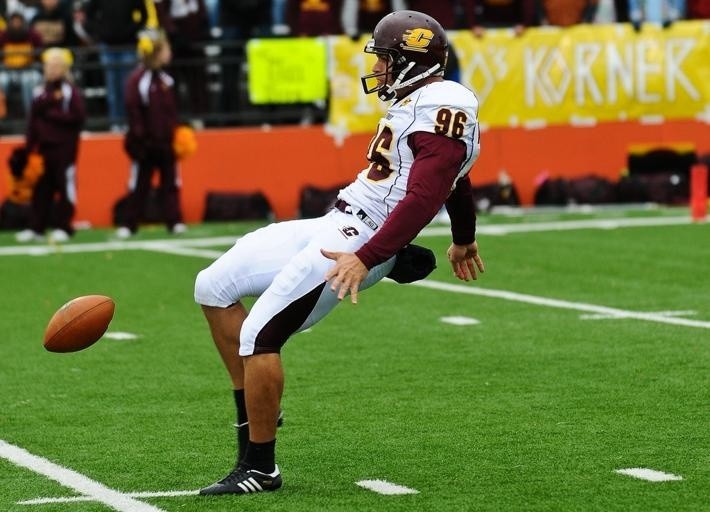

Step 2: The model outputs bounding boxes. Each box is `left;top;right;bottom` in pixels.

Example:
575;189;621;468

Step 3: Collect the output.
111;29;188;238
15;46;88;242
193;9;487;497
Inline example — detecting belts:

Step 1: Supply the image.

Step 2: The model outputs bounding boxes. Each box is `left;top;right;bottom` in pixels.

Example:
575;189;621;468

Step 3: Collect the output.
333;197;379;234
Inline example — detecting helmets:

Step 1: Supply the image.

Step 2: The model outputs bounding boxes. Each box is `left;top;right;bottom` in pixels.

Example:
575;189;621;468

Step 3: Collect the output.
367;8;451;90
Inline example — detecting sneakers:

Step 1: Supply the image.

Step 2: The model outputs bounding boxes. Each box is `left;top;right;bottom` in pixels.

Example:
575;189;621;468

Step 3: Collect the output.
14;222;186;244
194;410;286;496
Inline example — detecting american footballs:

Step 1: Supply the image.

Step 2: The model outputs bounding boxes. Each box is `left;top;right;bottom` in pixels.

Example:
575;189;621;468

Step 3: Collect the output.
44;295;115;353
